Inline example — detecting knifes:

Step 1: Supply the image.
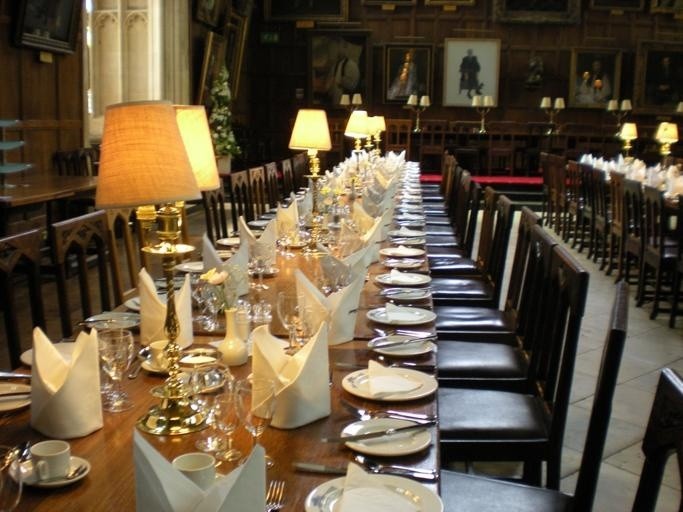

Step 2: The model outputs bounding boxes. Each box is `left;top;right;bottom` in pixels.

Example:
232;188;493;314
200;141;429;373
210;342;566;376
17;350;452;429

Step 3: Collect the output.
319;421;435;442
293;460;435;480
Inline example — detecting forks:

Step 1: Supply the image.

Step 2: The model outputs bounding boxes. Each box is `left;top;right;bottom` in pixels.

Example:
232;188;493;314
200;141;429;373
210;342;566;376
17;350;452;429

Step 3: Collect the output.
262;478;285;511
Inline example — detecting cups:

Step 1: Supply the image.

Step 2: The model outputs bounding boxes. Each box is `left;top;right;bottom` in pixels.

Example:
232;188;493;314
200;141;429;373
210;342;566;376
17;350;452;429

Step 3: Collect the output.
172;453;214;488
29;440;72;481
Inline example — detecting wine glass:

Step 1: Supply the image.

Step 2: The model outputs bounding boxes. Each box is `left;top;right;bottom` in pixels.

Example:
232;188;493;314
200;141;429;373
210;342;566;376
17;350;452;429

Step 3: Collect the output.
236;377;275;471
188;363;227;452
0;154;437;411
211;393;242;461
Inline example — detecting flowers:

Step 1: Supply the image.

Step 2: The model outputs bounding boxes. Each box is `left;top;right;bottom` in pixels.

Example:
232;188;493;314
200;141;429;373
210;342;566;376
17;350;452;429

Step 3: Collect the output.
207;60;243;160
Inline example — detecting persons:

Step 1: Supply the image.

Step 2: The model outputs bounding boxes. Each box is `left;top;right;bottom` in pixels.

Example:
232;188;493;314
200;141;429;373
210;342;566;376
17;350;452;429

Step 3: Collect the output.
392;52;418;96
652;55;680;105
577;59;611;105
459;47;484;99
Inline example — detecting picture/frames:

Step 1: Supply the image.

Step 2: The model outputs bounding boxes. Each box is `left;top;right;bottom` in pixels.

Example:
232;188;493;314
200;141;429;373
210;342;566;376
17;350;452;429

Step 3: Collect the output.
195;0;252;104
383;43;435;105
567;48;623;110
300;29;371;109
441;37;498;108
633;39;683;115
20;0;82;55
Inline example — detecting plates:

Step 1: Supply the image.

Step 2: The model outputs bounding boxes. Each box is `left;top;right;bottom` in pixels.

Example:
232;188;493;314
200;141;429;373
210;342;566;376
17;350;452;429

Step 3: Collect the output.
7;453;90;488
340;417;432;456
303;471;445;512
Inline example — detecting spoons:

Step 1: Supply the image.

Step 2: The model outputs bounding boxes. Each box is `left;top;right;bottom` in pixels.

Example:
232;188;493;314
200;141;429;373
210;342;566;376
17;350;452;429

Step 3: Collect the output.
0;441;30;472
354;453;435;474
338;399;437;425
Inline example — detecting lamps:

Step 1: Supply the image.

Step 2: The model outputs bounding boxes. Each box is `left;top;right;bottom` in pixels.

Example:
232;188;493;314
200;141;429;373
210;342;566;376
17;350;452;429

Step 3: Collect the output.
407;94;431;133
339;94;363;111
539;96;565;123
472;96;495;134
608;99;632;133
